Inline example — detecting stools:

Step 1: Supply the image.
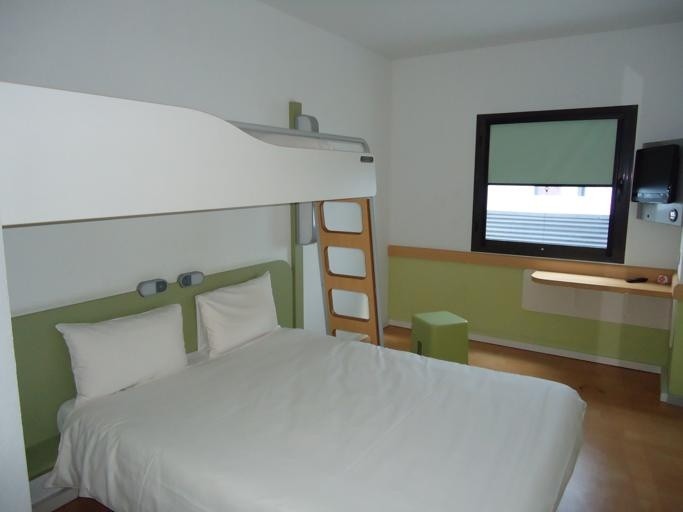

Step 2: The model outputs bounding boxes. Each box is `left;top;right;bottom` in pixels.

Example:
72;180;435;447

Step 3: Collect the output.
410;310;469;366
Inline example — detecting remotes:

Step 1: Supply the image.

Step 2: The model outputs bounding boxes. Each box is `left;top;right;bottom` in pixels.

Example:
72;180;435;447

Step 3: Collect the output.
627;276;650;283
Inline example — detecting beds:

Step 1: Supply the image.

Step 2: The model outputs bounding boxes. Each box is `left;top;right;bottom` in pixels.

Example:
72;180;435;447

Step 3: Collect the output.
0;79;377;229
10;261;586;511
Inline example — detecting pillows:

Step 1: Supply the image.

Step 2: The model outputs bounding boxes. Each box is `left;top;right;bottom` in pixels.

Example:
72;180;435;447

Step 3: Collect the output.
195;270;278;361
54;303;188;408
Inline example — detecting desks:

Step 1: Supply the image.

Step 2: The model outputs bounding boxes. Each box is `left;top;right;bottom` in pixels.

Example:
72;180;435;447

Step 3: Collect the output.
521;268;683;349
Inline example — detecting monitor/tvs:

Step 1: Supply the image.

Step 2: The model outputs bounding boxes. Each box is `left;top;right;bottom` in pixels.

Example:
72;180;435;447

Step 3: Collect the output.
630;144;681;205
